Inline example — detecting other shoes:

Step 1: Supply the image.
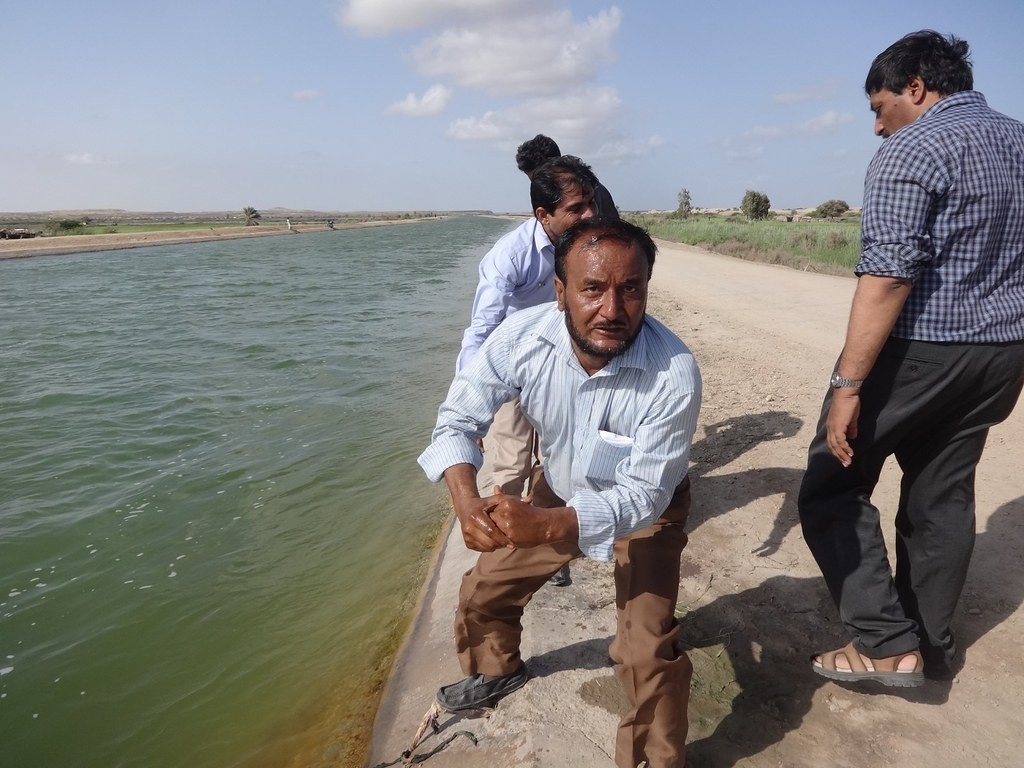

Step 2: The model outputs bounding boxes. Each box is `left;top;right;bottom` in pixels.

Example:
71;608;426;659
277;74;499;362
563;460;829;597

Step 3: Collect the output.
436;659;528;710
546;566;566;585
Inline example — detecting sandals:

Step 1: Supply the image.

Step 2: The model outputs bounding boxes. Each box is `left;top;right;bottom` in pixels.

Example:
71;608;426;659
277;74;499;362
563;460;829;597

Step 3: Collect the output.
810;641;924;687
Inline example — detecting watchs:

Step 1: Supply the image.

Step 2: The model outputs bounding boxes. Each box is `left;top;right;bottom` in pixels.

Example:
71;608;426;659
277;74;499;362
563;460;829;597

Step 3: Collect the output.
831;371;864;390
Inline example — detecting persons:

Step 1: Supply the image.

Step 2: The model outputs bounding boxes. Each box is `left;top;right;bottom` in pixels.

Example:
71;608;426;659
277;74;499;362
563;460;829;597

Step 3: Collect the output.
453;155;602;585
286;217;293;230
416;217;704;767
795;28;1024;688
516;137;623;223
